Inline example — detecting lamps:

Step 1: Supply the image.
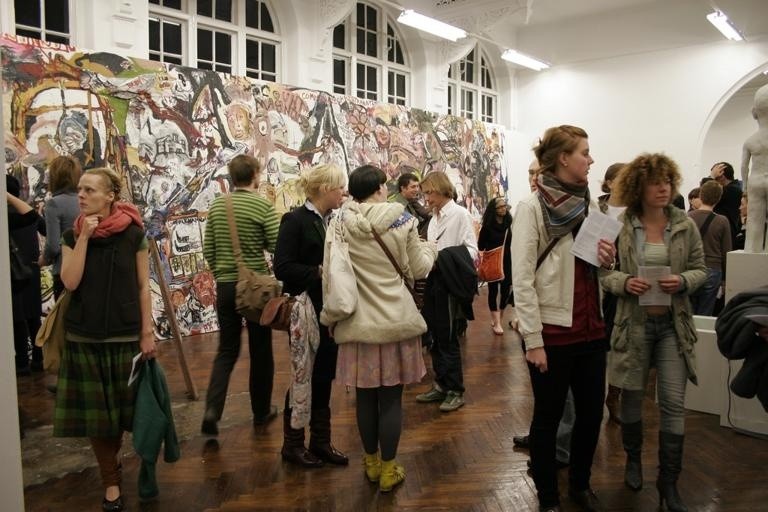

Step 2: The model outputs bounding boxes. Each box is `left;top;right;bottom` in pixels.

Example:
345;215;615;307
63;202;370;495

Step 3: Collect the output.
396;9;467;42
706;10;746;41
499;48;551;72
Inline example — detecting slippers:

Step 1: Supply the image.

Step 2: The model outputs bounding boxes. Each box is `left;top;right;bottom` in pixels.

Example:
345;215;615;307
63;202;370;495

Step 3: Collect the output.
491;320;504;336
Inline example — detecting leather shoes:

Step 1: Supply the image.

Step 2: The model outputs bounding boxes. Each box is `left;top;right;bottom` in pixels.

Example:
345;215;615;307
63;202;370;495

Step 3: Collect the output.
104;487;121;512
309;443;350;467
572;485;602;511
281;442;321;467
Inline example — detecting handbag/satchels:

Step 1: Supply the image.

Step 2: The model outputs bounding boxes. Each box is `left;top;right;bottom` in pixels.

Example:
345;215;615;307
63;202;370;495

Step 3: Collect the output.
479;246;505;283
603;290;616;345
236;268;284;325
258;292;296;331
34;290;68;374
320;240;360;327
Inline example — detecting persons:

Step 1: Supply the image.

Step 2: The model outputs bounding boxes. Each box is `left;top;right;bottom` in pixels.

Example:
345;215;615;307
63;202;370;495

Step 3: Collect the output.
740;83;768;252
480;192;515;335
5;171;46;384
39;148;89;304
199;152;280;438
271;159;480;495
513;126;768;512
53;162;173;509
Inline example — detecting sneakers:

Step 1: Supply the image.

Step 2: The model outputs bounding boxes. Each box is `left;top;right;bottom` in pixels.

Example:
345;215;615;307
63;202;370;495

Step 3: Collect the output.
202;410;221;437
439;390;466;412
416;387;445;401
45;382;56;395
15;353;45;372
254;405;276;424
512;435;565;471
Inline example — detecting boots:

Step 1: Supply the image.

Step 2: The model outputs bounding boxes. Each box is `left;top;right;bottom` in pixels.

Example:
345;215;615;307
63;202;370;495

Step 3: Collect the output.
621;419;643;493
605;383;622;425
364;451;405;493
657;429;688;511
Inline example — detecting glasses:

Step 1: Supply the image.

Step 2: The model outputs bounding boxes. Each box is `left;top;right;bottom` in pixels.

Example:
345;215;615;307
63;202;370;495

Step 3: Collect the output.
497;204;508;209
